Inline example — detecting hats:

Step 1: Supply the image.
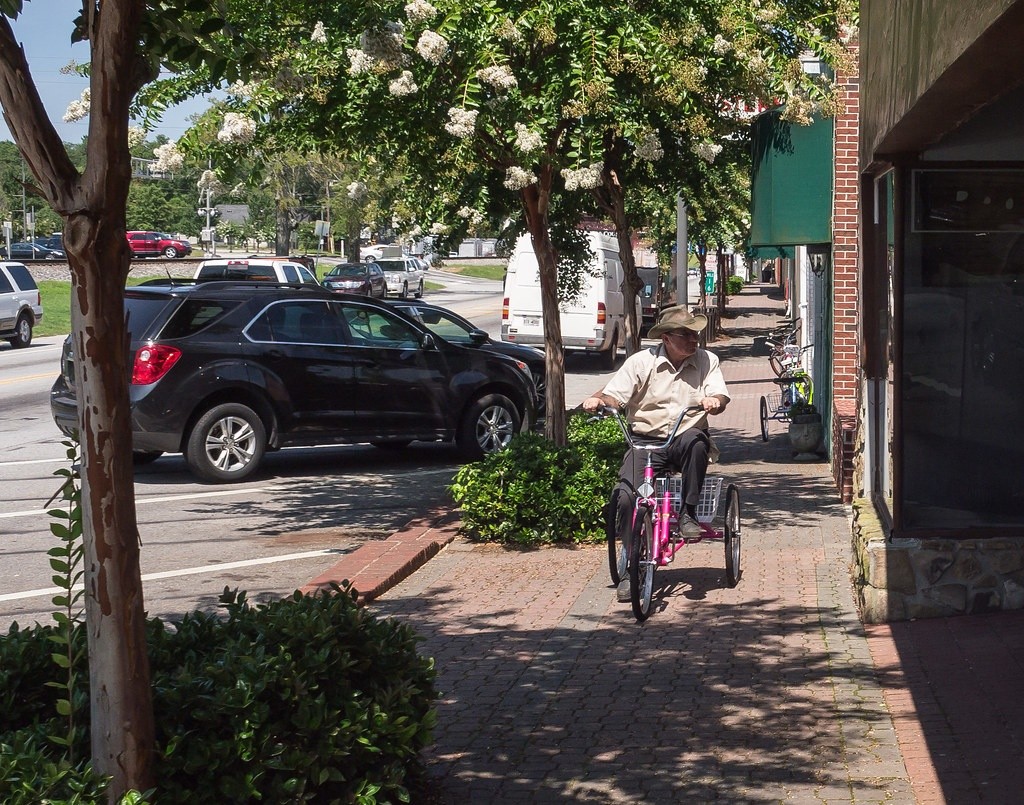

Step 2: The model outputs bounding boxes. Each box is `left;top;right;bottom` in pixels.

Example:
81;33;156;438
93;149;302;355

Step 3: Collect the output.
647;304;708;339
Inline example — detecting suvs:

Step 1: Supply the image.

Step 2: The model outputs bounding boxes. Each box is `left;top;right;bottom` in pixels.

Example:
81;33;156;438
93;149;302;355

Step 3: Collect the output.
126;231;192;258
191;257;321;289
372;259;425;298
36;239;62;249
51;279;539;481
0;262;43;349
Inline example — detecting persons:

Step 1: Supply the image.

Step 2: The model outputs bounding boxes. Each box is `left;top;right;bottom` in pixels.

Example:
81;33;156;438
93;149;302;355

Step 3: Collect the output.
583;304;730;600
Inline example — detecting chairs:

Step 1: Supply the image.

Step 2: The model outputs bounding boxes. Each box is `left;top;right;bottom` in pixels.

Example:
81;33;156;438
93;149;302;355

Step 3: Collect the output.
299;313;324;342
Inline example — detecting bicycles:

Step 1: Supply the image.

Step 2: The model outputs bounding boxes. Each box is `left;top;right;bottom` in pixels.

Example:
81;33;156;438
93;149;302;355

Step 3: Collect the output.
759;317;815;440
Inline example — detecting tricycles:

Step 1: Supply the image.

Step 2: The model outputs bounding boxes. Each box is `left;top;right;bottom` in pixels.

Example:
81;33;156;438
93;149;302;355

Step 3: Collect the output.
586;404;743;620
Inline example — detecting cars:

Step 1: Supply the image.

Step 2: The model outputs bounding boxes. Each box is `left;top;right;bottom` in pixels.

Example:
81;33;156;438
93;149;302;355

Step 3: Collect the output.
320;262;387;298
0;243;67;260
341;298;546;418
360;244;416;262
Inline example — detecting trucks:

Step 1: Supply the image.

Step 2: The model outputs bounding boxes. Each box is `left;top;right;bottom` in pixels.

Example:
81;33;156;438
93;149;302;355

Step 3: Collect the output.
631;244;677;323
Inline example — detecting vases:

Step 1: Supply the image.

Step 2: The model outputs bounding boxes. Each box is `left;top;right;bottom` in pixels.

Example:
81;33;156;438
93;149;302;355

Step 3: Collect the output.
791;414;825;462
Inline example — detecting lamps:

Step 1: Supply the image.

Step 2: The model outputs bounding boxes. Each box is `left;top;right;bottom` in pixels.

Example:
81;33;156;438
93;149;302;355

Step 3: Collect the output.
807;244;829;277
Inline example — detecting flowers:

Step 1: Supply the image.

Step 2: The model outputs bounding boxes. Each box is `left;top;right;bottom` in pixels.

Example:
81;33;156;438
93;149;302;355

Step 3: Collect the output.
786;398;818;420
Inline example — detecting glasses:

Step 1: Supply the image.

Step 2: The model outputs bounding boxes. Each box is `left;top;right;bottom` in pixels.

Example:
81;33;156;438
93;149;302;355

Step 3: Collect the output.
669;330;700;337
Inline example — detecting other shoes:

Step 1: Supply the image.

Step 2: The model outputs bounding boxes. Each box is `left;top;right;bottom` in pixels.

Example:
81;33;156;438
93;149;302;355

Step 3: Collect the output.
617;573;631;601
677;509;702;538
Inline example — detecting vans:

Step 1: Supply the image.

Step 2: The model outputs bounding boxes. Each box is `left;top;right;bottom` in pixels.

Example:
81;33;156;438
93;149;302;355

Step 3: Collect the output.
501;229;643;369
